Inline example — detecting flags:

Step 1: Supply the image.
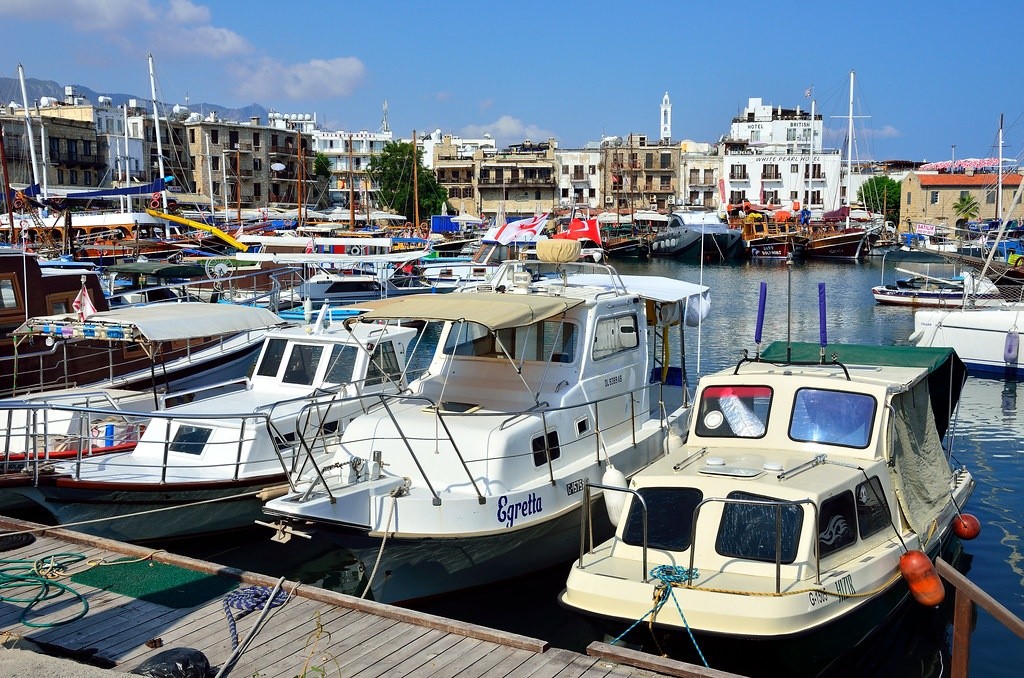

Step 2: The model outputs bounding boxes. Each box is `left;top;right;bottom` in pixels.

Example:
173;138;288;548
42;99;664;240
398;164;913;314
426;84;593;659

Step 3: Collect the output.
552;219;602;246
306;240;314;250
72;284;97;322
232;225;243;240
482;212;548;245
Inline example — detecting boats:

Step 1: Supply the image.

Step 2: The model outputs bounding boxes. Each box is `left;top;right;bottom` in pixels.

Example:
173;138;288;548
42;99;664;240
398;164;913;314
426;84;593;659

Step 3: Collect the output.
557;279;978;678
0;47;1024;569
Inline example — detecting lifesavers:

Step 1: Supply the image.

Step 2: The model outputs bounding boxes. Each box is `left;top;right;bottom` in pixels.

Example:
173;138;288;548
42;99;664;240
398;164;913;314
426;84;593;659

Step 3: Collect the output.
404;222;412;230
421;233;426;238
152;192;160;200
284;220;291;226
14;200;23;208
351;247;359;255
150;200;159;208
421;222;428;230
